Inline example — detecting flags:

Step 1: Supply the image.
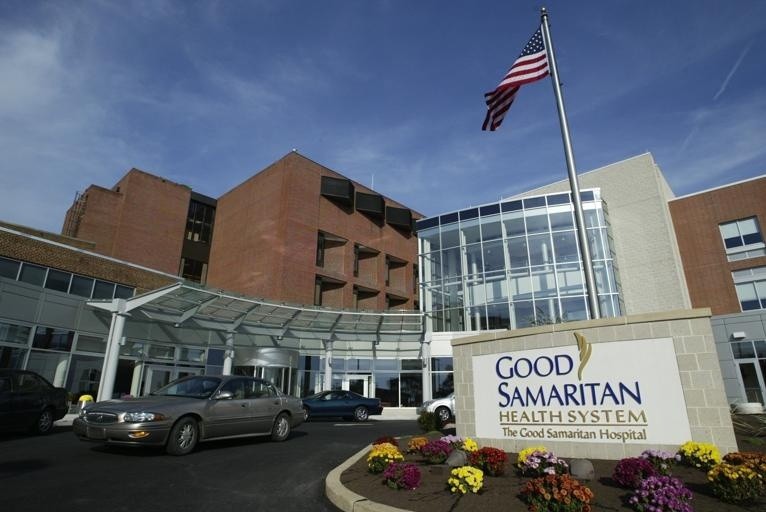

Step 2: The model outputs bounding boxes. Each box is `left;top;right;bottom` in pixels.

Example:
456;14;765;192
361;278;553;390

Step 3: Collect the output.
481;23;554;132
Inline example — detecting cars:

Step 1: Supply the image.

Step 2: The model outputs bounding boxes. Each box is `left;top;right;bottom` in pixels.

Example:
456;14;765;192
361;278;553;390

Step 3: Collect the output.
0;367;70;434
300;389;383;422
72;375;306;455
417;392;455;424
